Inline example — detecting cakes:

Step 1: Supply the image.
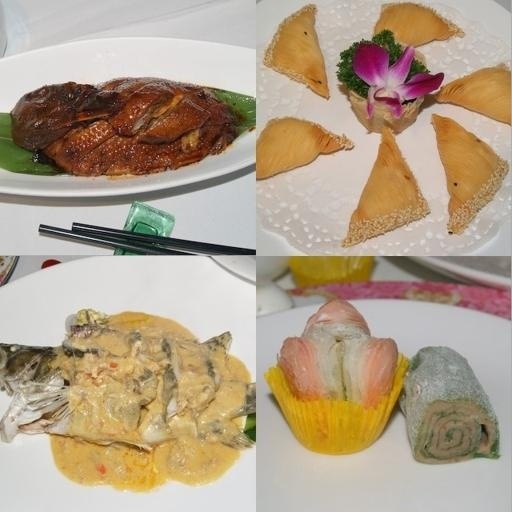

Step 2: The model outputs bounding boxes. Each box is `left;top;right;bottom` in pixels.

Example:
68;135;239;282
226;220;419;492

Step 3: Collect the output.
397;347;499;465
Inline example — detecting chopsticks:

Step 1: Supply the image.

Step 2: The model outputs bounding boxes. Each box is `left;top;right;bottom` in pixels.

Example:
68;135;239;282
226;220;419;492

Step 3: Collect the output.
39;222;255;255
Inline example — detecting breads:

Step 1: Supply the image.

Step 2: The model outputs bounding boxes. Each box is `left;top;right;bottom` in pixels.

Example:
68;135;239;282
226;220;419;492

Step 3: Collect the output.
432;113;509;234
341;126;430;248
266;300;410;456
374;2;465;47
264;4;330;99
434;67;512;125
256;118;354;179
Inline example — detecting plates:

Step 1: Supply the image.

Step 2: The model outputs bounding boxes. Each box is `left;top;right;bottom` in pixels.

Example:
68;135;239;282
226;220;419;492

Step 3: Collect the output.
0;256;256;512
256;297;511;512
0;35;256;199
